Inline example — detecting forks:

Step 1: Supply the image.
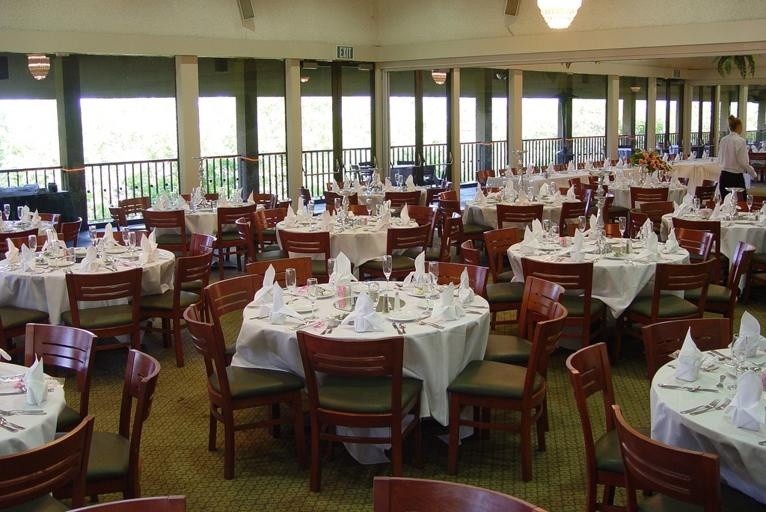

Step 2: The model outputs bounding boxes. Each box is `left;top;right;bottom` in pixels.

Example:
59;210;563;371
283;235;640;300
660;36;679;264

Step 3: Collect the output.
658;350;762;416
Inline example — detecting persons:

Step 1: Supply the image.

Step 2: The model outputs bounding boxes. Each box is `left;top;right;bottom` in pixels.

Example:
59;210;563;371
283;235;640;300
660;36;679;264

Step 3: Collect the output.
717;116;760;201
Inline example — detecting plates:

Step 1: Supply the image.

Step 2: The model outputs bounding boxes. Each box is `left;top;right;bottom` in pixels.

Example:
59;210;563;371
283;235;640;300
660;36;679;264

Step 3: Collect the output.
532;244;561;251
0;388;26;395
298;221;316;225
286;279;445;334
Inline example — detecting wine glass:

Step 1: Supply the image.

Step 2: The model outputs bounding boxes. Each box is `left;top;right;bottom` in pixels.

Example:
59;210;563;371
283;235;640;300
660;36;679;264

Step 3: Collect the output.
27;226;137;274
663;149;710;164
363;174;404;194
286;268;296;306
328;258;336;290
690;190;766;224
306;277;319;322
485;180;557;204
543;216;658;263
18;206;23;229
306;196;392;230
383;255;393;293
4;204;10;226
613;170;671;188
422;273;434;315
428;261;440;299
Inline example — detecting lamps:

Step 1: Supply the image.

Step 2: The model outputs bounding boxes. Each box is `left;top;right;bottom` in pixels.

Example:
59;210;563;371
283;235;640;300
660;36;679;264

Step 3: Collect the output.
26;54;52;82
430;69;449;86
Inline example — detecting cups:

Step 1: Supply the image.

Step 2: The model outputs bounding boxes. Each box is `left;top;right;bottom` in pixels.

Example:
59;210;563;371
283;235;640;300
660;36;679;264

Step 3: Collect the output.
169;189;244;213
731;333;748;367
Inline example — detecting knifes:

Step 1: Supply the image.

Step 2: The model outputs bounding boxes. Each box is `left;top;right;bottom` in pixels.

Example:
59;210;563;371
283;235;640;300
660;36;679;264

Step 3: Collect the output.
0;408;46;415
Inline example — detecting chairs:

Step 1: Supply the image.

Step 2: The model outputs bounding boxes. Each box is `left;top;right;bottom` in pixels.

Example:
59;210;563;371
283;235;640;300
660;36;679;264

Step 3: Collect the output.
1;140;765;281
23;323;98;432
202;273;262;367
640;316;732;380
564;341;650;510
295;330;423;492
56;349;162;500
618;255;717;346
78;495;188;511
184;304;306;480
128;248;214;368
446;299;569;483
61;268;144;353
480;276;565;438
374;476;544;511
520;258;606;351
685;241;756;340
461;239;523;331
1;303;52;349
3;415;95;510
610;405;764;511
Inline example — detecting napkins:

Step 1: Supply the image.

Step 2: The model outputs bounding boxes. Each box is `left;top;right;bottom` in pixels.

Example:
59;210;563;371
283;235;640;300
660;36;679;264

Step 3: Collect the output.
675;326;706;383
401;251;436;291
339;290;386;333
430;280;465;322
22;353;49;405
247;263;274;307
724;370;764;431
454;266;474;305
734;310;761;356
328;252;359;287
259;280;304;326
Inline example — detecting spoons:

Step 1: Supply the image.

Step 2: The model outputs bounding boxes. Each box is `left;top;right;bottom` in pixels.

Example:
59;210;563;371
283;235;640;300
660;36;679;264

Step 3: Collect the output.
0;416;25;433
